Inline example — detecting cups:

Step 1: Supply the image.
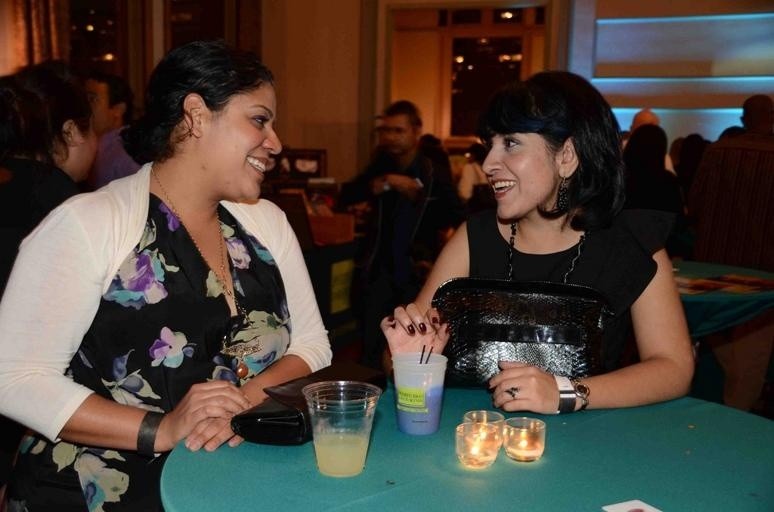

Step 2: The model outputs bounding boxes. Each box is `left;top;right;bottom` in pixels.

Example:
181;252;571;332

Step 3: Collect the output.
300;379;383;478
464;409;504;449
503;414;546;463
456;420;499;471
386;352;449;437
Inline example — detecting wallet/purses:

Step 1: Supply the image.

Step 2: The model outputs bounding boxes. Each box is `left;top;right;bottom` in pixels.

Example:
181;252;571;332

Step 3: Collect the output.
231;357;386;446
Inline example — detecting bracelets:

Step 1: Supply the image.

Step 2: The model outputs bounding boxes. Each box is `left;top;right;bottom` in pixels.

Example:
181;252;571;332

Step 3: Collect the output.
554;375;576;415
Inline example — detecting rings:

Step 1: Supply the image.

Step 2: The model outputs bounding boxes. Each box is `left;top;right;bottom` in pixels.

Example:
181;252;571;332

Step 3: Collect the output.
505;390;515;398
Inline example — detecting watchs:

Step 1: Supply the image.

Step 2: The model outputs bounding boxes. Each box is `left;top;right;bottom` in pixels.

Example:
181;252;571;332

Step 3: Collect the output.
573;378;590;411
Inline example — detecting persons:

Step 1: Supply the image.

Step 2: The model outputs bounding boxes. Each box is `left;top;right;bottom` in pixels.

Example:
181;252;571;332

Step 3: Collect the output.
330;98;455;370
0;39;334;510
622;93;774;271
381;67;695;415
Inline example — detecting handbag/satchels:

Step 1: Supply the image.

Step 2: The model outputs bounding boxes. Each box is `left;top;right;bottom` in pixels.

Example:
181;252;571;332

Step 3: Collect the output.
431;277;615;389
